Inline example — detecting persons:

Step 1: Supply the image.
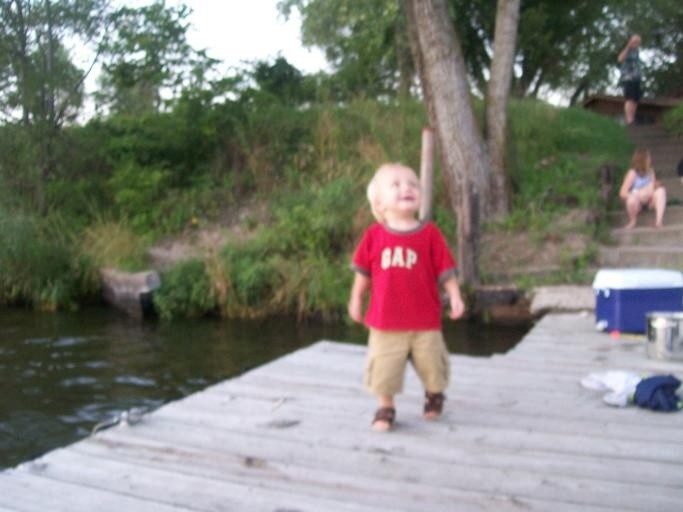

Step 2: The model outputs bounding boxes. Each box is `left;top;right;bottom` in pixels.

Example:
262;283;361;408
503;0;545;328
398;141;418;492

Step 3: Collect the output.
613;32;645;127
346;161;466;433
616;144;668;230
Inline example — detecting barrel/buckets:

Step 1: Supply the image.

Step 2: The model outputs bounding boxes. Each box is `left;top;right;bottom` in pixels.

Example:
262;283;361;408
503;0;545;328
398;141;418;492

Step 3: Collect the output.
645;310;683;362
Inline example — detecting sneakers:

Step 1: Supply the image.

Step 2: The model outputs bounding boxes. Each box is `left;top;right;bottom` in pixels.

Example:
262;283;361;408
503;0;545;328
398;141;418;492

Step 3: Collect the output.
423;390;444;419
371;407;395;433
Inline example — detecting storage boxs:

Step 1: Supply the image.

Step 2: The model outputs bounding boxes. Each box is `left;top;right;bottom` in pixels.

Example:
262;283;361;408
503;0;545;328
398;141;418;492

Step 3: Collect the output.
591;268;683;336
